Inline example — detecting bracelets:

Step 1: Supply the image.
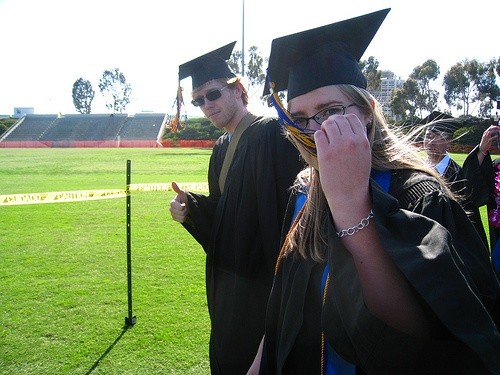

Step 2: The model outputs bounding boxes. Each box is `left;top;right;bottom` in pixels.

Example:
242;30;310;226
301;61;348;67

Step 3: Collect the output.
336;209;375;239
479;145;487;155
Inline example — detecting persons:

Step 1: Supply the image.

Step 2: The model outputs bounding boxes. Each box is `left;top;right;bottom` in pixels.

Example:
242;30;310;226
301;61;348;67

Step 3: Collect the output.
260;8;500;375
459;125;500;282
419;110;462;195
169;40;309;375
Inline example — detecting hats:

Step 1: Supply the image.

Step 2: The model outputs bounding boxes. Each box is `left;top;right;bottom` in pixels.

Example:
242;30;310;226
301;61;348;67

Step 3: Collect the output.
171;41;238;133
263;8;391;159
414;111;464;135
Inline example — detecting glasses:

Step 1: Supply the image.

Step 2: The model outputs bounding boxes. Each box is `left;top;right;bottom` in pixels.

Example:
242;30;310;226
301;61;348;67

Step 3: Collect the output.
191;85;228;108
292;103;356;131
